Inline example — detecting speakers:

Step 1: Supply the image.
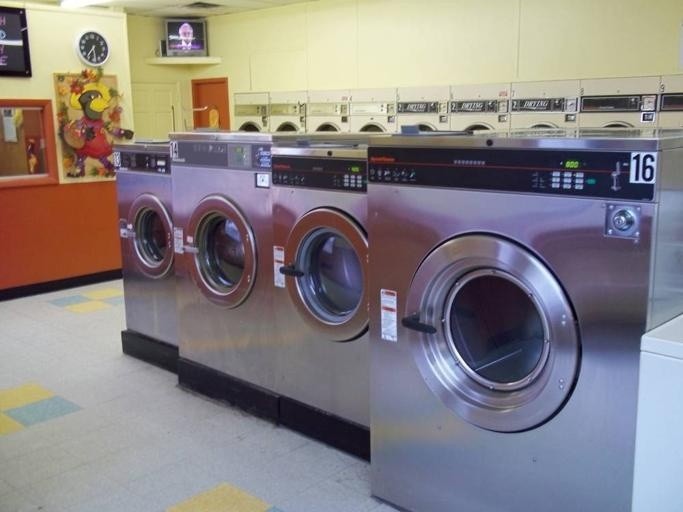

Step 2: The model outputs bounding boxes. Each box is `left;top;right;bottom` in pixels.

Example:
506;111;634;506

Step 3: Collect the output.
159;40;167;57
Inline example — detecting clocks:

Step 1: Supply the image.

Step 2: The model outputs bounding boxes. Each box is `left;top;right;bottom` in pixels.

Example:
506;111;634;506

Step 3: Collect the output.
75;31;111;67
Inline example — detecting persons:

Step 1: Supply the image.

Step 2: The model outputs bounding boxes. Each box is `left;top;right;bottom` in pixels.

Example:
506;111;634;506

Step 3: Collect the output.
169;23;200;49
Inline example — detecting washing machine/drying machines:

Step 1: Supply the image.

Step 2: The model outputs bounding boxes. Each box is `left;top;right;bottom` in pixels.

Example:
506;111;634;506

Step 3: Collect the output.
109;91;682;511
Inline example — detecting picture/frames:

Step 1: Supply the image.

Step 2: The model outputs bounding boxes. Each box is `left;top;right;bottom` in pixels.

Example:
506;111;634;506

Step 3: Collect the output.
0;98;59;189
50;66;126;186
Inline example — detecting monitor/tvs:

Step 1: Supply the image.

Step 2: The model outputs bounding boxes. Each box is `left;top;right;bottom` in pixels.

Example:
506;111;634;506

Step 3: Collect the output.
165;19;207;57
